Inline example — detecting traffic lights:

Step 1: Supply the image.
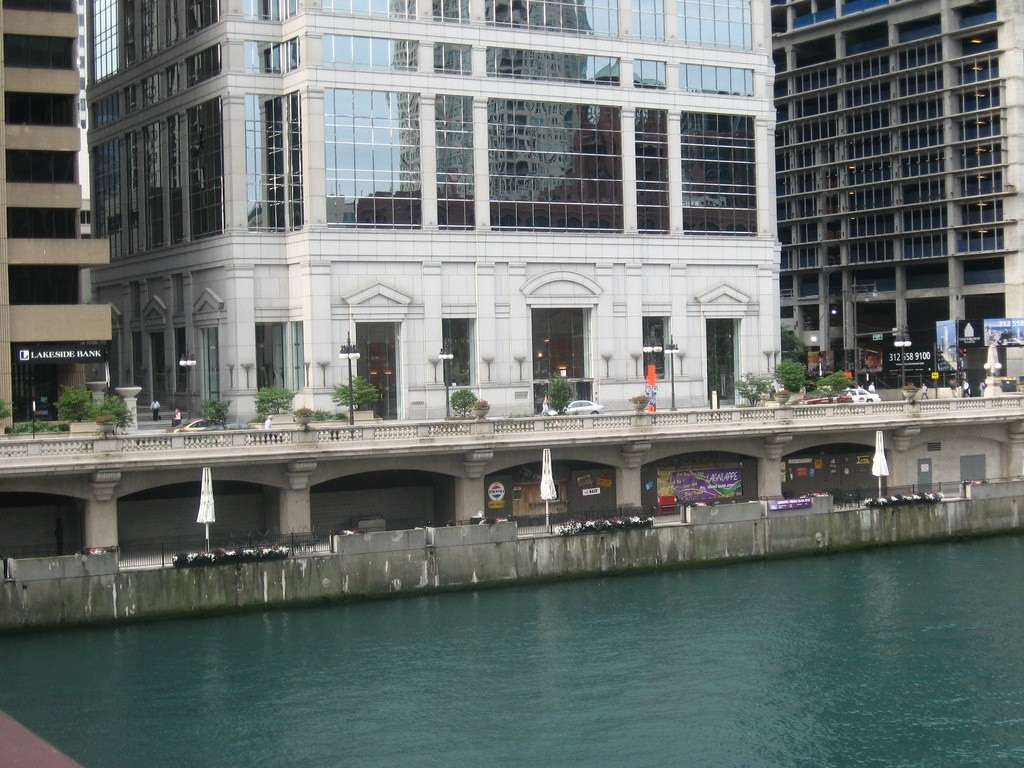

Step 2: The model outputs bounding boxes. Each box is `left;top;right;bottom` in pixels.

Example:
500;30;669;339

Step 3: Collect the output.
844;348;852;363
858;348;864;361
858;360;864;370
846;361;854;371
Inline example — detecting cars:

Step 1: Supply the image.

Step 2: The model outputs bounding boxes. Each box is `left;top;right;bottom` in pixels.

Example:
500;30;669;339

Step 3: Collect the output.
792;388;854;414
165;416;225;443
838;385;882;408
548;400;605;419
199;421;260;443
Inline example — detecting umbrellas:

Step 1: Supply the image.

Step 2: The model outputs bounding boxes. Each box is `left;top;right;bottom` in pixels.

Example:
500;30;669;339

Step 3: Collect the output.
871;430;889;498
196;467;215;550
539;448;556;532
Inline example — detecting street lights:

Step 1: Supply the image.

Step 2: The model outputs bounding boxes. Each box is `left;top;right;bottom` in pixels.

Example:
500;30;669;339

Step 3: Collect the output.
339;331;361;439
852;276;878;386
893;324;912;400
664;334;680;411
178;342;197;419
439;343;455;421
841;282;871;371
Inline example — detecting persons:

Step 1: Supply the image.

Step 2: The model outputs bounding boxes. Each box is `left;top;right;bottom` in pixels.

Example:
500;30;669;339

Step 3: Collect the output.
921;383;928;399
265;415;273;429
964;379;971;397
868;382;875;393
150;399;160;421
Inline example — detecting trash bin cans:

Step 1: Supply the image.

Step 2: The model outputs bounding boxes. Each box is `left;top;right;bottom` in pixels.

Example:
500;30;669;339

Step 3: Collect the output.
470;518;481;524
1002;379;1017;391
960;481;972;499
